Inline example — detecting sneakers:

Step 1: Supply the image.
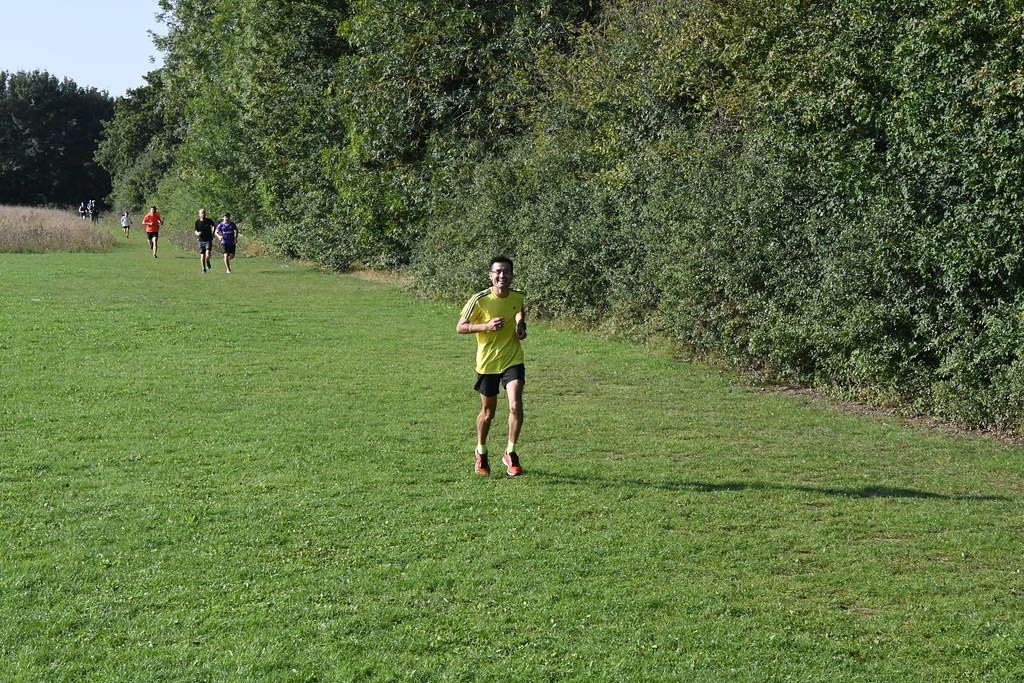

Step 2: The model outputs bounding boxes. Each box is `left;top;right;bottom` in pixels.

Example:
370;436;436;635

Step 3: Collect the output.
503;449;522;476
474;447;490;476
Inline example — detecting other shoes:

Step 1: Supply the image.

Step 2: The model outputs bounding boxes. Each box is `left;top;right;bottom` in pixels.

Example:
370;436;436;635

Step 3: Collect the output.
202;268;206;274
227;269;230;274
153;254;157;258
150;243;153;250
206;261;211;269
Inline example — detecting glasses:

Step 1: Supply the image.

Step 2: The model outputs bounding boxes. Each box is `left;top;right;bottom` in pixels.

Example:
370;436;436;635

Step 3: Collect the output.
491;270;512;275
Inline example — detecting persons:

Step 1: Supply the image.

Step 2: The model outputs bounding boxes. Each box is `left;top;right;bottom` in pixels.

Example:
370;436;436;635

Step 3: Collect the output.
120;211;133;238
214;212;239;274
194;209;215;273
142;206;163;258
457;255;527;477
78;199;99;224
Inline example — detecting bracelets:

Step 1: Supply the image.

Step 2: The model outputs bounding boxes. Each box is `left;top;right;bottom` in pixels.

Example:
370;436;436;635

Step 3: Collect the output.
469;323;472;334
